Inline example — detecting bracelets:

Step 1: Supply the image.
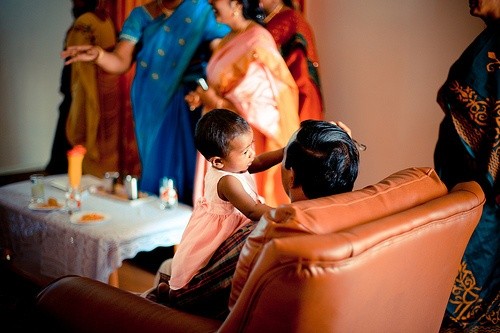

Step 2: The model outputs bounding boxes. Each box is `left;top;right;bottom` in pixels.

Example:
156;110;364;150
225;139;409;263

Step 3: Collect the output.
208;97;223;110
90;44;104;63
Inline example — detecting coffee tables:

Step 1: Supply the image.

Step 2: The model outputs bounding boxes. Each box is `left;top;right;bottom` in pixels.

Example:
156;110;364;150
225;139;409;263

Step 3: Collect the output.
0;173;193;293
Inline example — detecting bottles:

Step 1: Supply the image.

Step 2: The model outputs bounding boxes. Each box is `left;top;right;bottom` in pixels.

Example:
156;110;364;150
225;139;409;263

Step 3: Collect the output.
64;186;82;213
160;176;179;210
104;172;138;200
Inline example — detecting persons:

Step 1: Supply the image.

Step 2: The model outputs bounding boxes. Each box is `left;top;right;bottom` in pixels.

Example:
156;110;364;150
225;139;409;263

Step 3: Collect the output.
432;0;500;333
63;0;231;213
45;0;140;189
254;0;329;122
168;108;351;290
141;119;359;323
185;0;301;212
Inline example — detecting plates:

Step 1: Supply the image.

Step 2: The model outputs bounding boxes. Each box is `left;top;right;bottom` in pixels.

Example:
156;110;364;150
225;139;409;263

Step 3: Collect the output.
50;174;103;192
28;201;65;211
70;209;112;225
89;183;157;206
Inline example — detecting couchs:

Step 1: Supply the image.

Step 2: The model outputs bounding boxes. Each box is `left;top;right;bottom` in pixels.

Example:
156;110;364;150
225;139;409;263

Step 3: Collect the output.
12;167;487;333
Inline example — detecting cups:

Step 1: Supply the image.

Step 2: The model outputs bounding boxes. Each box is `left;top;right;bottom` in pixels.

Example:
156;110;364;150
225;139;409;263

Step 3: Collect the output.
30;174;46;205
